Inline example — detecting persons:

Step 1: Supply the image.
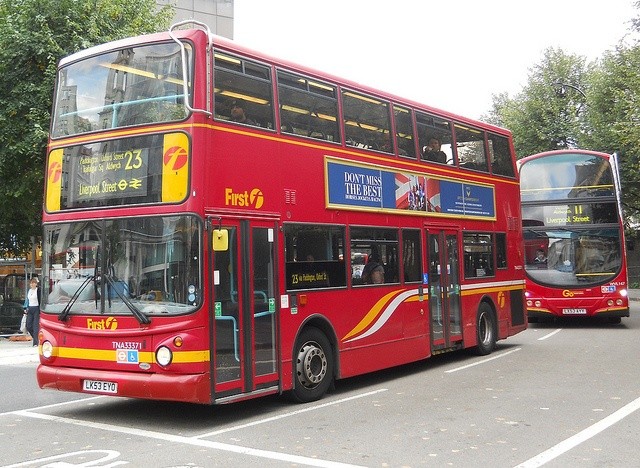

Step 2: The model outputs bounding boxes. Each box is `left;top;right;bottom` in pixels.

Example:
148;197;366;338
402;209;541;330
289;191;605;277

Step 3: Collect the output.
424;137;447;163
108;251;146;282
22;277;40;348
225;100;257;126
369;266;385;284
534;249;548;264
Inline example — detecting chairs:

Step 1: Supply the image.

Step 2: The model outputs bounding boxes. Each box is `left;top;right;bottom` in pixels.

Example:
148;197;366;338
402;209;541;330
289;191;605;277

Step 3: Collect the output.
307;129;327;140
419;151;477;169
281;121;295;134
333;135;355;146
363;141;379;151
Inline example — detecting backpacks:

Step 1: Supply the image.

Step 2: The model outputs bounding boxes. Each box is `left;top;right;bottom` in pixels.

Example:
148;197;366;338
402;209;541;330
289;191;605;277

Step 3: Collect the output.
365;265;379;284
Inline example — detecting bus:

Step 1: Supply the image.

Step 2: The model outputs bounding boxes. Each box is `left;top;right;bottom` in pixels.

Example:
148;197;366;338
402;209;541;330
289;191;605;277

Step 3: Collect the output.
514;150;631;325
37;21;529;403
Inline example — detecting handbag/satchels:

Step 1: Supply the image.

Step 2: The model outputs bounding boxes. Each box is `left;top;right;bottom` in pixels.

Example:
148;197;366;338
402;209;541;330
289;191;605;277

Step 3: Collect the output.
20;314;27;334
